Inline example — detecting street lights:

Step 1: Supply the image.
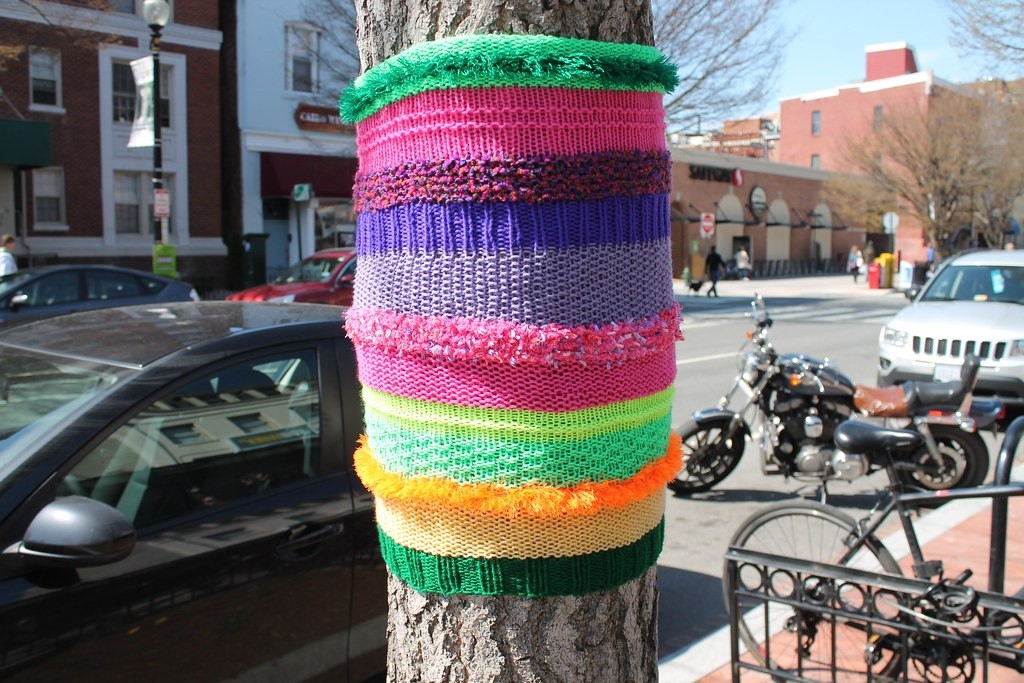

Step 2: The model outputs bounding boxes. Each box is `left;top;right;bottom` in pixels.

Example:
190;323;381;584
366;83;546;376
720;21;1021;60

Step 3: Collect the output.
142;0;171;243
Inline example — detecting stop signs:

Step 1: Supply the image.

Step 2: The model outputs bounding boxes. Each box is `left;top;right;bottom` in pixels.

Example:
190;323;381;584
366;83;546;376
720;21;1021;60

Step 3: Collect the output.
700;212;715;237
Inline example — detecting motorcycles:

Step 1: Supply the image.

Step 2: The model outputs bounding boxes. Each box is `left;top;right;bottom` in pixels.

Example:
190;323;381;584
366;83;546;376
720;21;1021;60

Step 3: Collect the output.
669;291;1024;508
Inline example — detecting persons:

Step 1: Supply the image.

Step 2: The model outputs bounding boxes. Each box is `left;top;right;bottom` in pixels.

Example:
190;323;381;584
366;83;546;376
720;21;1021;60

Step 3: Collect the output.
846;244;863;282
926;241;936;265
733;245;750;281
863;241;877;266
1;232;25;299
702;245;726;298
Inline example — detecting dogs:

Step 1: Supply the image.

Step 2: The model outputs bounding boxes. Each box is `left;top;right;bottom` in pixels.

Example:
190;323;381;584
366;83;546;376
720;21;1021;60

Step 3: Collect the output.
688;281;704;296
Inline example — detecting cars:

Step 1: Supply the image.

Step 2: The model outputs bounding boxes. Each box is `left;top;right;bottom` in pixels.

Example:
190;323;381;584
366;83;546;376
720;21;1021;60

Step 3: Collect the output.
0;263;200;324
0;299;388;683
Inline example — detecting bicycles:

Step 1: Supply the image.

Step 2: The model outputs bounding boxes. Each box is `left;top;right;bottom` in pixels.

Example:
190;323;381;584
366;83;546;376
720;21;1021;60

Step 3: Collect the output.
721;419;1024;683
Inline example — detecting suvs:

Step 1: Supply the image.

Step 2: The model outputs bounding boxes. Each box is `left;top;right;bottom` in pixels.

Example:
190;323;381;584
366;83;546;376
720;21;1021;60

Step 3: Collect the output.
225;246;357;306
874;247;1023;426
